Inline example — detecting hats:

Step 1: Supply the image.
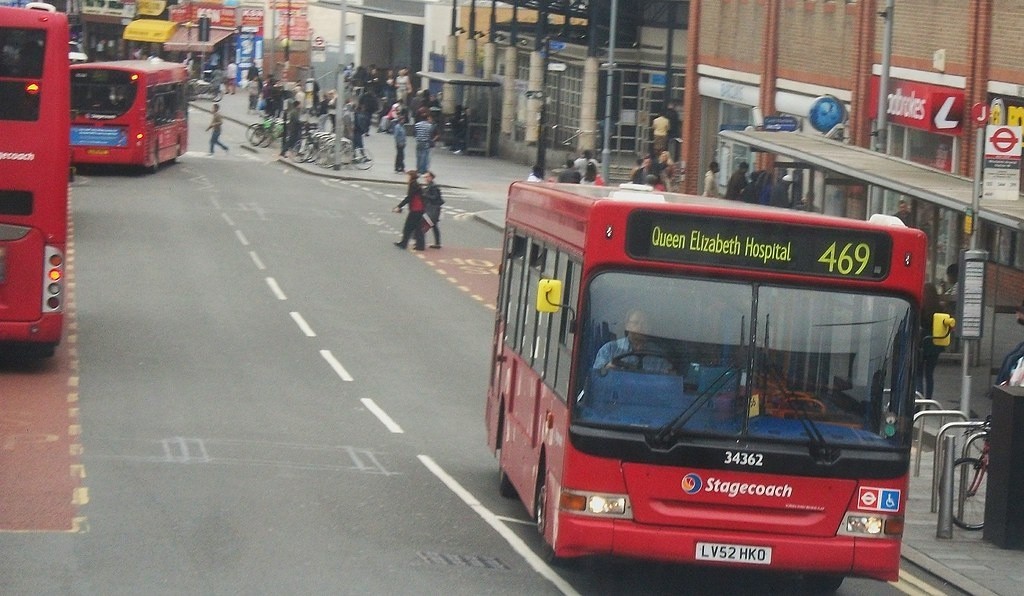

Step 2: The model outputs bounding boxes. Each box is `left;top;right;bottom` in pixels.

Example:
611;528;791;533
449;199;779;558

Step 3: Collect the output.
783;175;790;182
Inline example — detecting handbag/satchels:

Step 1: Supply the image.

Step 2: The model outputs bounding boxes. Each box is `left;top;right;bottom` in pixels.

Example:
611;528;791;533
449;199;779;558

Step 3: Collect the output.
439;199;444;204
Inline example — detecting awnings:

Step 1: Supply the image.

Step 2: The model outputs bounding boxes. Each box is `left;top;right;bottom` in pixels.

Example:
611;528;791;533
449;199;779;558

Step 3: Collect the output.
122;17;178;42
718;128;1024;230
163;23;236;51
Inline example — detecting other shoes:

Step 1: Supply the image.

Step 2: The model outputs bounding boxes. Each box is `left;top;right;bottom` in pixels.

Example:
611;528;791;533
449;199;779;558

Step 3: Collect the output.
429;245;442;249
413;247;425;250
394;242;407;249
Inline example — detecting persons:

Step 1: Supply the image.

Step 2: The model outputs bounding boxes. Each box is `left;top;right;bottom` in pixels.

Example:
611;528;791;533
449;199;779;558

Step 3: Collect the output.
913;282;943;414
701;161;721;199
414;171;442;249
941;263;959;312
93;37;221;80
224;57;237;95
246;61;468;175
206;103;229;156
592;306;674;376
393;116;407;172
893;200;915;228
526;101;776;206
392;170;425;251
986;302;1024;399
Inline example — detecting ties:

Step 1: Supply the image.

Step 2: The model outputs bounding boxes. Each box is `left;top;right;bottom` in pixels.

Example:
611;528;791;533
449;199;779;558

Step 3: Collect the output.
637;355;644;370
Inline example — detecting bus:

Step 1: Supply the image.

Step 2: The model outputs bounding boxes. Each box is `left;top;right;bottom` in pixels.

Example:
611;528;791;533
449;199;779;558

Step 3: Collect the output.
484;178;956;596
0;4;73;354
69;58;194;174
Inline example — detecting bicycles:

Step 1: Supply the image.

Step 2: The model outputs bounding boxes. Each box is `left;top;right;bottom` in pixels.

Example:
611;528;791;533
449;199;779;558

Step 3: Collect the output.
191;76;223;103
939;413;991;531
243;116;374;171
